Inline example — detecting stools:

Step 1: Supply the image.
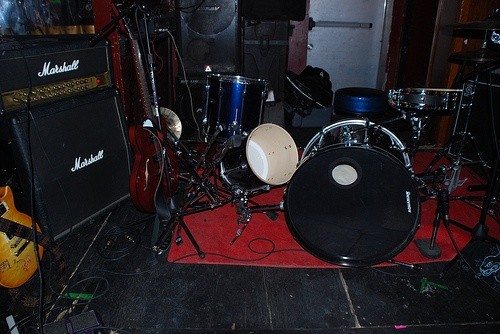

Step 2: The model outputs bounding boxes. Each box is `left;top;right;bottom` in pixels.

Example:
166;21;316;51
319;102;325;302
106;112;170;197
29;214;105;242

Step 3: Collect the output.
334;88;389;146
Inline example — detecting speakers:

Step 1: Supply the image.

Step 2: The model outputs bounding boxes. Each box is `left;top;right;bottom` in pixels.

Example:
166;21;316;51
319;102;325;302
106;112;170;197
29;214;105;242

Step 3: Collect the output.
0;87;134;243
177;0;241;77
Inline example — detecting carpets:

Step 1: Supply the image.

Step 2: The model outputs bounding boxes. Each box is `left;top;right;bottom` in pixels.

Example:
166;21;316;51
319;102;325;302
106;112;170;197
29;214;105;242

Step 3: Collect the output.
167;142;500;268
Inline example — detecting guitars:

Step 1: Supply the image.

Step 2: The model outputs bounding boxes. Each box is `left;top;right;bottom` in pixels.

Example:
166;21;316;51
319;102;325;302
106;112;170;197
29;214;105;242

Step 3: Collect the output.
0;185;44;289
110;0;179;214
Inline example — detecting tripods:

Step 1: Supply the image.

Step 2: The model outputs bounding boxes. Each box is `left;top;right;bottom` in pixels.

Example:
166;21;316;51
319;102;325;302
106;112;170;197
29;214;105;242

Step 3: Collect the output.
409;31;500;270
89;0;279;259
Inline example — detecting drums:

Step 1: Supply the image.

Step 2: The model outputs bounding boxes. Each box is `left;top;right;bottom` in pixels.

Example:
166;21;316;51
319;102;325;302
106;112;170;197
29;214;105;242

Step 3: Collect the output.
219;122;299;193
280;119;421;267
387;87;463;119
286;76;326;111
201;73;269;149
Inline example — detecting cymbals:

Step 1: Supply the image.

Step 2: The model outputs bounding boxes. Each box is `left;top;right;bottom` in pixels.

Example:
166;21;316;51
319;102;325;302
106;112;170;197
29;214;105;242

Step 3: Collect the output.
159;107;182;140
444;15;500;31
448;50;500;65
